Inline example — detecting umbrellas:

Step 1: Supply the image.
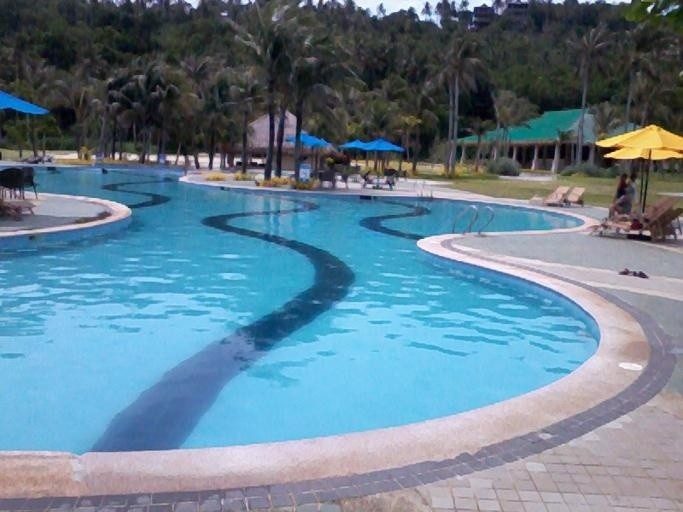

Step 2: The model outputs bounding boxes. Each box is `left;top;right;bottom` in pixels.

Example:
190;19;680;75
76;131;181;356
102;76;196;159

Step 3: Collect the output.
602;147;683;206
594;123;683;213
0;87;51;116
287;129;405;189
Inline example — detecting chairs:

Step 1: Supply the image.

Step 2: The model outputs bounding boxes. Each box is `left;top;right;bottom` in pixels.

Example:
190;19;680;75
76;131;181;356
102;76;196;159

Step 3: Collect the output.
543;186;586;206
601;196;683;243
0;165;39;201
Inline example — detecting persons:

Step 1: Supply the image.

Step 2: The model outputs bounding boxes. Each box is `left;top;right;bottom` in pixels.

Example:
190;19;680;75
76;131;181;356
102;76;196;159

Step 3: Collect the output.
385;164;399;191
608;172;640;218
